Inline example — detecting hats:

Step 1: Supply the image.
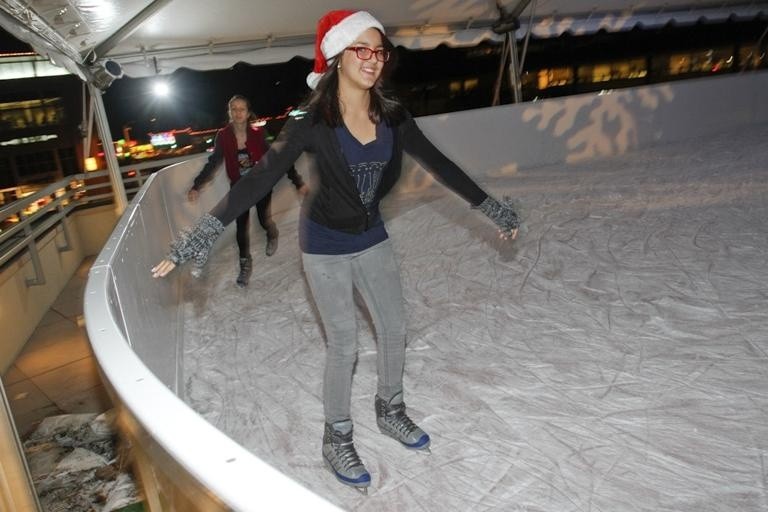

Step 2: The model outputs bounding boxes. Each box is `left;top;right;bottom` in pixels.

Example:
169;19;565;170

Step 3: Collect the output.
305;9;386;91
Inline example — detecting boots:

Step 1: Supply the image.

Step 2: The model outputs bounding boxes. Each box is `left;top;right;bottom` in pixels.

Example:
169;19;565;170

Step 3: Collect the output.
375;390;430;450
265;224;279;257
236;256;253;284
321;419;371;488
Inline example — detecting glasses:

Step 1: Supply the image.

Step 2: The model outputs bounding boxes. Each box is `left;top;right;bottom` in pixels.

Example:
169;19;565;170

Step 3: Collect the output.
345;46;391;62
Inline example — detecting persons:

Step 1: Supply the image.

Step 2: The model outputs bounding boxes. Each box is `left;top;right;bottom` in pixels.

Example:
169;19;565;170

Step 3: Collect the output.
148;6;523;493
186;94;310;285
